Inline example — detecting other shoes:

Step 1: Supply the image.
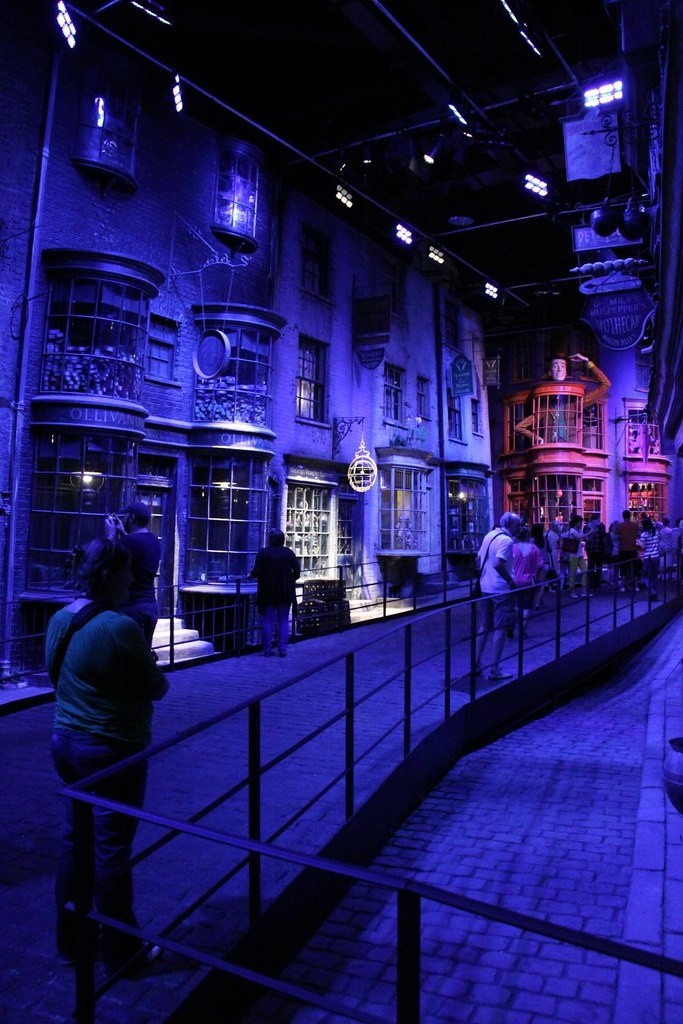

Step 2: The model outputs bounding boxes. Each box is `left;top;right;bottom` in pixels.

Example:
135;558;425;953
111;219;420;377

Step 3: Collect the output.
582;594;593;596
507;629;513;639
569;594;578;599
58;953;152;979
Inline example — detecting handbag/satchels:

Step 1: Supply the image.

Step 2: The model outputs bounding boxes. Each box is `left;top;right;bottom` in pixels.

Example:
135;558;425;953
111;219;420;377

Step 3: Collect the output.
562;529;579;553
471;583;482;600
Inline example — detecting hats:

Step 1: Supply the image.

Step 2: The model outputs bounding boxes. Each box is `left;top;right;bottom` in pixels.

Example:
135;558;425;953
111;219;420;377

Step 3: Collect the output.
120;502;150;521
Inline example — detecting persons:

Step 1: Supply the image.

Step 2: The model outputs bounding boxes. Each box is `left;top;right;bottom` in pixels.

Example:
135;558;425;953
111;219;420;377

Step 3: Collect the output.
474;510;524;681
45;537;167;980
102;501;162;650
246;529;301;659
516;509;683;638
515;348;612;528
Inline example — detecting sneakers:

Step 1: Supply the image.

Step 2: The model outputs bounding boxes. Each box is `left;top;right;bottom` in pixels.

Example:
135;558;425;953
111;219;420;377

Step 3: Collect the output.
488;668;513;680
